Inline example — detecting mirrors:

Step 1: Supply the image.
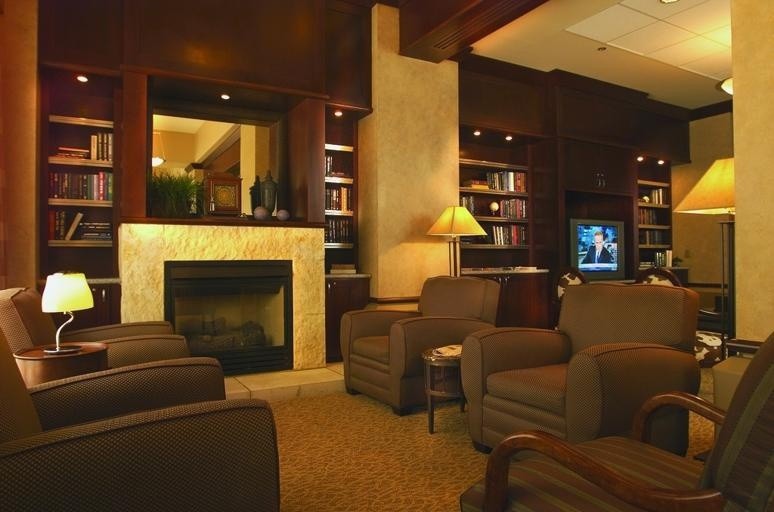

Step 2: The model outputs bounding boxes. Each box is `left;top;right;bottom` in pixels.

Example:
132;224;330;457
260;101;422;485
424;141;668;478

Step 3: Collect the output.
142;106;295;224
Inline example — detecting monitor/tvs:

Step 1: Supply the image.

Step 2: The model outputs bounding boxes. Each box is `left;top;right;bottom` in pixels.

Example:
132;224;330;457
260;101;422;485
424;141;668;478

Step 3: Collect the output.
569;218;625;281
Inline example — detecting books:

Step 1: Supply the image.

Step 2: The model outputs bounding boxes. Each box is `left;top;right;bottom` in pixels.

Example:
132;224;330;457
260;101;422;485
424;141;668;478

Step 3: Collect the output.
325;155;358;274
638;188;673;268
460;170;527;247
47;131;114;240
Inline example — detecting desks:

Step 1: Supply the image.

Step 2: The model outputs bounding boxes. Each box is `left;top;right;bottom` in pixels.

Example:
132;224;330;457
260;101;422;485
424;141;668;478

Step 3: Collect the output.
424;346;464;435
11;343;113;390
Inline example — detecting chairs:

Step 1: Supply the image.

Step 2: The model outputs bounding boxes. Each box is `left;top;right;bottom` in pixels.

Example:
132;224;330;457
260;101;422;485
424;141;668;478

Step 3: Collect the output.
1;277;190;366
554;267;586;301
456;274;700;456
337;274;501;416
1;333;284;512
456;323;773;512
637;269;728;372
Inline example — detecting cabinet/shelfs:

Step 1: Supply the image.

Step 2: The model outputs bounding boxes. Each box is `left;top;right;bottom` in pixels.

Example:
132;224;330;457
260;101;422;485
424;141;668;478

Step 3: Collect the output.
639;263;691;285
531;141;558;271
318;98;372;278
48;276;122;331
459;126;535;270
632;149;678;266
324;278;371;363
459;267;550;330
44;78;114;279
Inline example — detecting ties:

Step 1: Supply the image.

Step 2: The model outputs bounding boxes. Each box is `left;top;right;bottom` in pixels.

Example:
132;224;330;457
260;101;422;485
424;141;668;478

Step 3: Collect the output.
596;251;600;263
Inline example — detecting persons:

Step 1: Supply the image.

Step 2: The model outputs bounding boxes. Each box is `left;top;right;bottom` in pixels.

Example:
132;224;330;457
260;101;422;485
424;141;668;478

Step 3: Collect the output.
582;232;615;263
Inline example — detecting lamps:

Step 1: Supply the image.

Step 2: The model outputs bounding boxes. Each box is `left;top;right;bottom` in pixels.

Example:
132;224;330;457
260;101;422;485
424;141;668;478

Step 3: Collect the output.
428;206;487;276
670;154;737;215
35;270;97;355
720;76;734;97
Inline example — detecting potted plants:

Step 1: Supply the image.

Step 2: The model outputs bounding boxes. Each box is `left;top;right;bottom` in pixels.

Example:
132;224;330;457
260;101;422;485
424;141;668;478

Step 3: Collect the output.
146;172;205;218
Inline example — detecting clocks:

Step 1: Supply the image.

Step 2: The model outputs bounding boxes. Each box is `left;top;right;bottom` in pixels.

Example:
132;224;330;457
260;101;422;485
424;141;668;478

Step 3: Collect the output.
206;171;243;218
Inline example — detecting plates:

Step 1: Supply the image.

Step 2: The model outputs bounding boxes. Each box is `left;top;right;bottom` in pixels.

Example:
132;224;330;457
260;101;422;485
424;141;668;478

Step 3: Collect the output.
431;344;465;358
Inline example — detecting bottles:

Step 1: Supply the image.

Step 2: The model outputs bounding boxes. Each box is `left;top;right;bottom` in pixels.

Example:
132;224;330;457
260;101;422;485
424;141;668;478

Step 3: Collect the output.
259;169;277;221
249;175;262;214
